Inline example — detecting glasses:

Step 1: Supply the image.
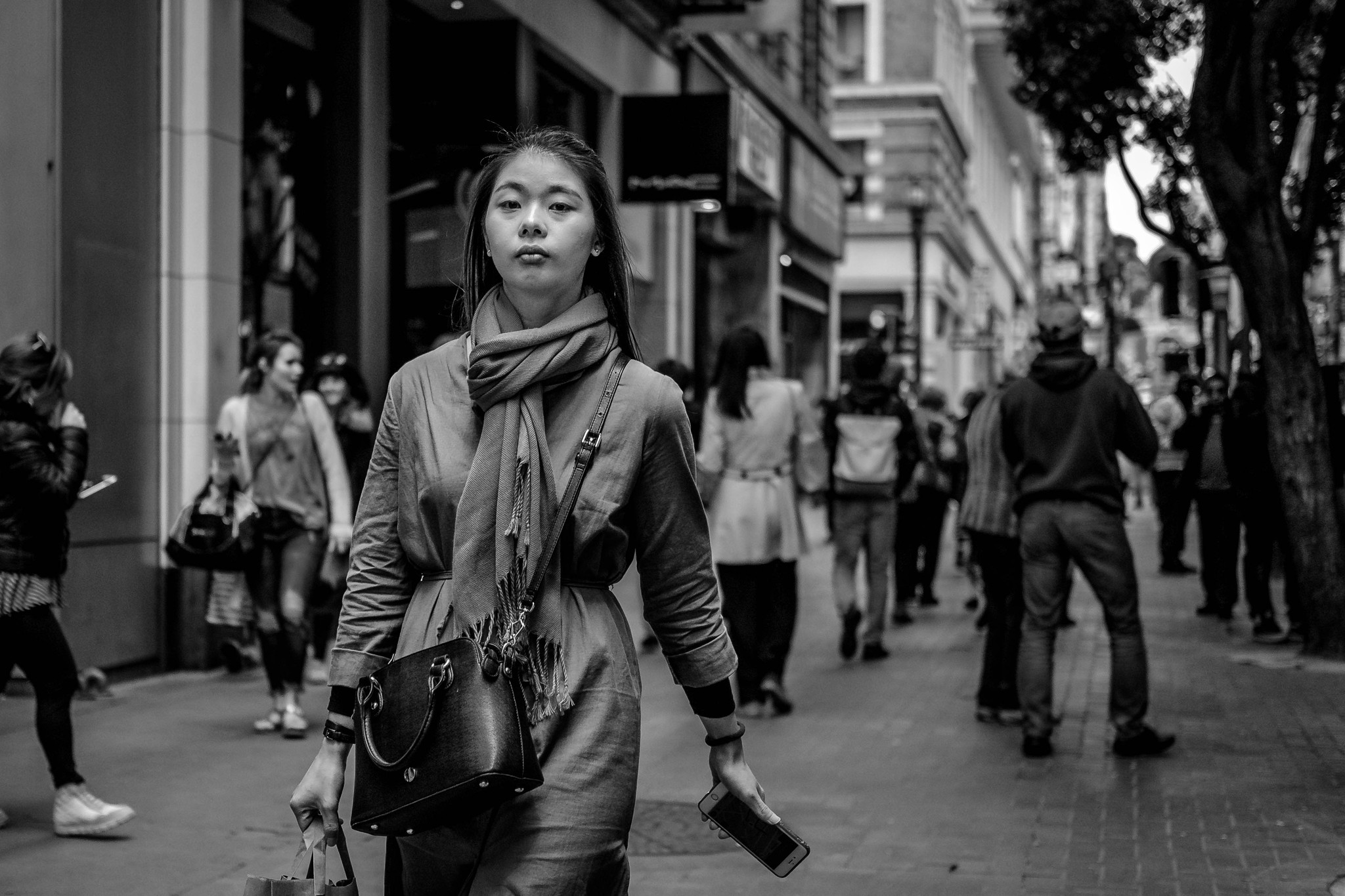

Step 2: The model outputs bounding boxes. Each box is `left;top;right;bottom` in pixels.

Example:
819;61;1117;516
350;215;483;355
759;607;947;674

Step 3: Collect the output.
30;331;53;354
319;354;348;365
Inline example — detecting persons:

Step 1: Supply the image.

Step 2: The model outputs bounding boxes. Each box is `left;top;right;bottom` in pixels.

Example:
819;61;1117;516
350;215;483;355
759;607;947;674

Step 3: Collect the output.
207;327;374;740
1003;294;1177;763
0;333;133;832
1130;376;1305;639
290;130;779;896
639;328;1076;724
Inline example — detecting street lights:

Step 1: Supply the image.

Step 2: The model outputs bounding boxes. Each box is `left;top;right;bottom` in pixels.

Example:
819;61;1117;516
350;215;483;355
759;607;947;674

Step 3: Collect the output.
893;173;936;378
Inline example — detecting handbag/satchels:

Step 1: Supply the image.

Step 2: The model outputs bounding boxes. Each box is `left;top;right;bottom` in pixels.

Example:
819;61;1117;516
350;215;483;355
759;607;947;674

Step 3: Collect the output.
350;637;544;835
246;817;361;896
164;474;263;573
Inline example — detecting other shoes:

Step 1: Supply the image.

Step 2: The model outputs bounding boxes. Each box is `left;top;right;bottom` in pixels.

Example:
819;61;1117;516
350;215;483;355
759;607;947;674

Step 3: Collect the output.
1196;603;1233;621
52;785;135;836
839;610;888;661
1252;620;1285;643
255;709;281;733
1158;556;1197;573
1113;722;1175;756
1022;738;1058;760
745;678;792;717
281;705;309;737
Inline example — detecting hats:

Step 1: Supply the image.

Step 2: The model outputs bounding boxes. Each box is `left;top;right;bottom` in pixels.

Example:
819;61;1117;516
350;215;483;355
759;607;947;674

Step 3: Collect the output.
1037;302;1089;342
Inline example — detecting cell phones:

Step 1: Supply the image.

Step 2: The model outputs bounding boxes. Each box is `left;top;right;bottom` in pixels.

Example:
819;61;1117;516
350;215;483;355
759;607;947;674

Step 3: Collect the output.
698;780;810;878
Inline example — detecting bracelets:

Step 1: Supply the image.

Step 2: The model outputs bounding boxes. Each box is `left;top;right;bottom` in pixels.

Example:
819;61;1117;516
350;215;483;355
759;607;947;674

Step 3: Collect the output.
322;719;357;744
703;720;746;746
